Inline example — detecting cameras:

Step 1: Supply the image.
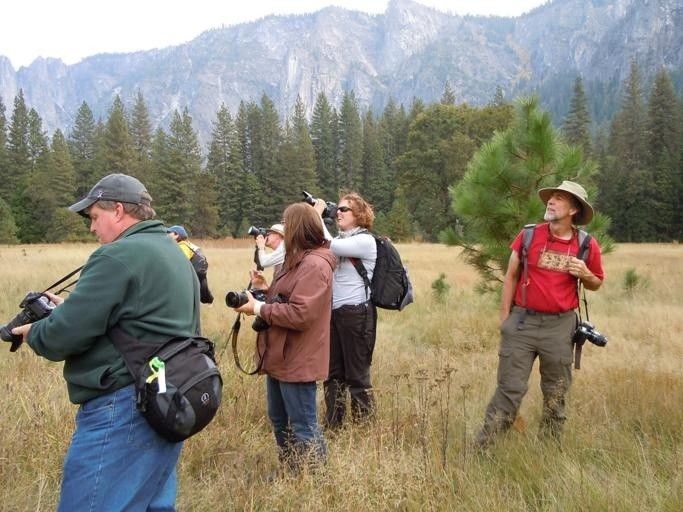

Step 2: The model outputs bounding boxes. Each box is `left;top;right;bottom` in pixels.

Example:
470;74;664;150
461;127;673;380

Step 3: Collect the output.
248;226;267;248
302;190;337;225
0;291;58;352
573;322;607;347
226;289;266;308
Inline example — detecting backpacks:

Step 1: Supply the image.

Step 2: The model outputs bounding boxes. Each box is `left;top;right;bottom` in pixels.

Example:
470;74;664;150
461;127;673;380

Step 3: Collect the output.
345;228;408;311
189;252;213;303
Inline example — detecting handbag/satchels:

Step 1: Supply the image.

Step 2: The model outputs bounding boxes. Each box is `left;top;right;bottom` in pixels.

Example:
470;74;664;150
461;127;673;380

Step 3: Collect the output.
135;337;223;444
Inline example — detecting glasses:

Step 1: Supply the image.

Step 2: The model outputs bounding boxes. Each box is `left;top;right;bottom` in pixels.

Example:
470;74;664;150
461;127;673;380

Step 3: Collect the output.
337;206;352;213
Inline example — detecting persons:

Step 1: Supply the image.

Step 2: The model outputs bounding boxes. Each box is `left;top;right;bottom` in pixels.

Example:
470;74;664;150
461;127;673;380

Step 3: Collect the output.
9;174;201;512
313;194;377;440
475;179;605;449
165;224;199;260
256;222;286;281
233;201;339;484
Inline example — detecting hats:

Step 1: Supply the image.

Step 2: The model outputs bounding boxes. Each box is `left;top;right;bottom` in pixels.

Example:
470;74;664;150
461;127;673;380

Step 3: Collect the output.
399;274;413;310
537;180;593;225
264;223;283;237
68;173;152;219
167;225;187;241
